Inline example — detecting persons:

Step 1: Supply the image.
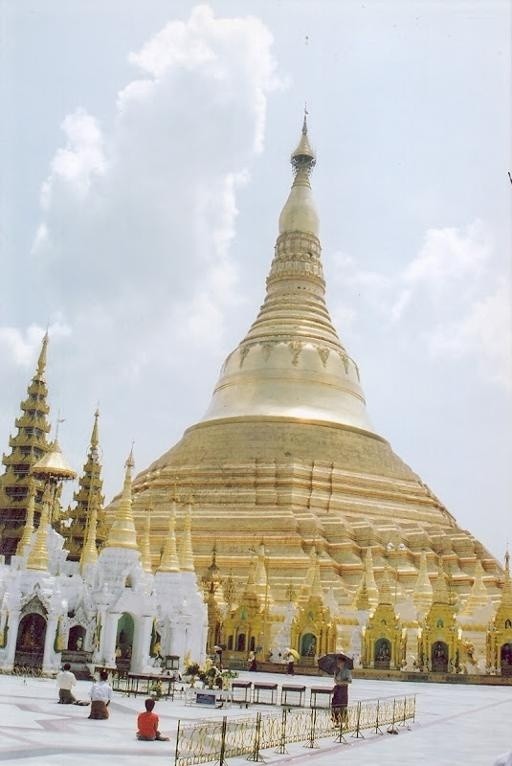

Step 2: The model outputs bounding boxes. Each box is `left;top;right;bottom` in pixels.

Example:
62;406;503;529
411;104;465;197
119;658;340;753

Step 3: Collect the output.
331;655;352;729
137;699;170;741
248;650;257;671
56;664;77;704
287;652;295;675
88;672;114;719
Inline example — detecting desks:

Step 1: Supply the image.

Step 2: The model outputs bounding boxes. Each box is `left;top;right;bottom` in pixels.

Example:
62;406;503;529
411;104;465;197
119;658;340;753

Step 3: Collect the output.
309;685;333;710
252;681;278;707
149;673;176;704
164;653;181;671
128;671;150;698
231;680;252;705
280;683;306;708
93;665;128;692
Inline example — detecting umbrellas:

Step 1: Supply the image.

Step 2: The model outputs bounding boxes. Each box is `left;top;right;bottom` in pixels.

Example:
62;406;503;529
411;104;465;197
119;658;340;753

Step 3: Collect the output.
288;648;300;660
317;654;354;675
